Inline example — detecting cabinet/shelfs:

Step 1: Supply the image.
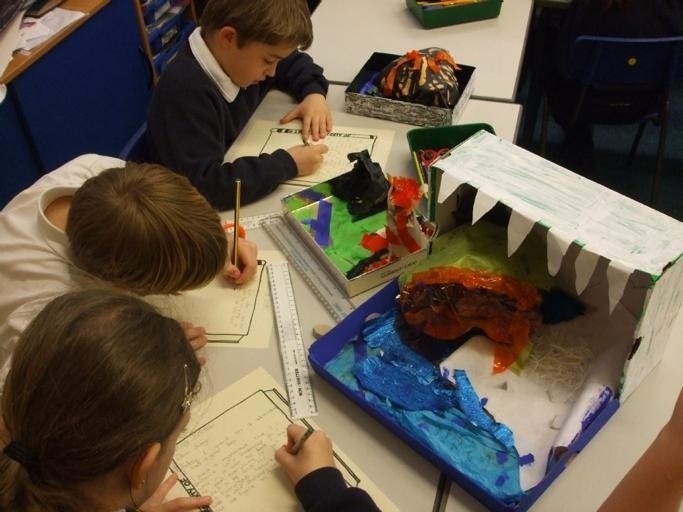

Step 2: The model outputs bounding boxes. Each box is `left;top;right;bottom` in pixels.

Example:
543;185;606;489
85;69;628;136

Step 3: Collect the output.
136;2;197;89
11;3;151;177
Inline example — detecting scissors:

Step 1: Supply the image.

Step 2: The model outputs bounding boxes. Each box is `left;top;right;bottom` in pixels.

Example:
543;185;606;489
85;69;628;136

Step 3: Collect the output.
421;148;450;174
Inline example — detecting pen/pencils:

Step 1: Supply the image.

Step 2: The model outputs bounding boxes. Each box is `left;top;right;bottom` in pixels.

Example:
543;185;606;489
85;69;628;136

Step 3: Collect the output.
303;139;309;146
232;179;241;290
289;428;314;454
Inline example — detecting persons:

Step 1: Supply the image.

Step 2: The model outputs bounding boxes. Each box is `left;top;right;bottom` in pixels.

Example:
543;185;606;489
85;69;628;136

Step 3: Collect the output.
145;0;332;212
0;153;258;397
0;287;383;512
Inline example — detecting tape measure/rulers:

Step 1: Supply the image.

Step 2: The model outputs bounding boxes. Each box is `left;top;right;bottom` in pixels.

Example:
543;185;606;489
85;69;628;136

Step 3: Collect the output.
226;212;356;418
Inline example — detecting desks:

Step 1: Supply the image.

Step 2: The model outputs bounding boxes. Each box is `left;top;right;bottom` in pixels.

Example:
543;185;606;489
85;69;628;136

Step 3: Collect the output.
145;85;678;508
2;1;106;89
300;2;533;103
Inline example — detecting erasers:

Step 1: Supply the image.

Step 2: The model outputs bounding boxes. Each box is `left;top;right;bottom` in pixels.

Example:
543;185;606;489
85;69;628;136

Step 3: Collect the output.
313;324;332;340
